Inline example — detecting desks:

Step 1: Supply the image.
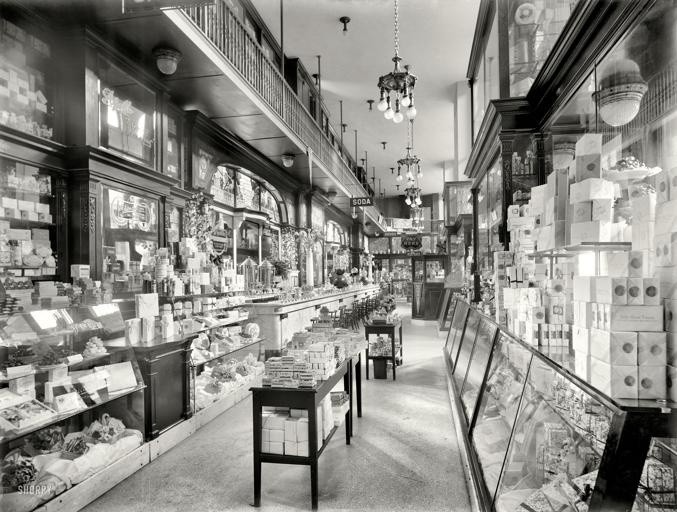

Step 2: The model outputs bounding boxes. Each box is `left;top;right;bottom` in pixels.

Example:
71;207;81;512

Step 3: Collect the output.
250;315;403;510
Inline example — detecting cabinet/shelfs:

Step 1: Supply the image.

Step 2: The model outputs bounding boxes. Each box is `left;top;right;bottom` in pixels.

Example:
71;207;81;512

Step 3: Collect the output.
0;303;148;509
171;290;267;415
439;287;677;512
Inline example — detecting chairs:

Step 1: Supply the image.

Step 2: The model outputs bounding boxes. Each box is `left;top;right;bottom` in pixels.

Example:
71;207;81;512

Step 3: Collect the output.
310;293;380;340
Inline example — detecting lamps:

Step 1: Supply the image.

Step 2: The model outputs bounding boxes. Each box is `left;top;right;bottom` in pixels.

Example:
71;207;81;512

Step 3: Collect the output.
154;49;183;76
281;154;295;168
328;192;337;202
592;82;650;130
377;0;423;227
